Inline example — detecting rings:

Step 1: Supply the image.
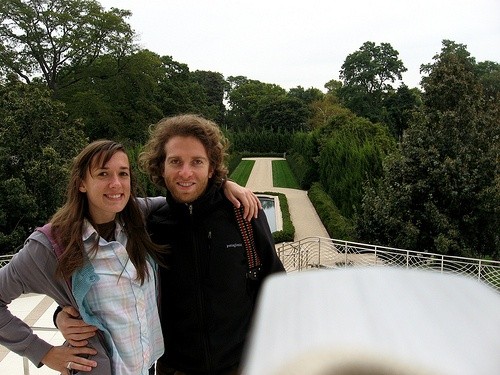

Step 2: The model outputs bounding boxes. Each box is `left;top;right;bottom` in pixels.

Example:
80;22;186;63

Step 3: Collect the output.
67;361;71;369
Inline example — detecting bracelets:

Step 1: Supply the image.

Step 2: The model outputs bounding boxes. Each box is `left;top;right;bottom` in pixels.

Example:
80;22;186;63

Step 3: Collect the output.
53;305;64;328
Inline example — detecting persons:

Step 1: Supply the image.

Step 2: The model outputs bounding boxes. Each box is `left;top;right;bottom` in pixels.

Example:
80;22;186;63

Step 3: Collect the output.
53;114;288;372
0;139;263;375
